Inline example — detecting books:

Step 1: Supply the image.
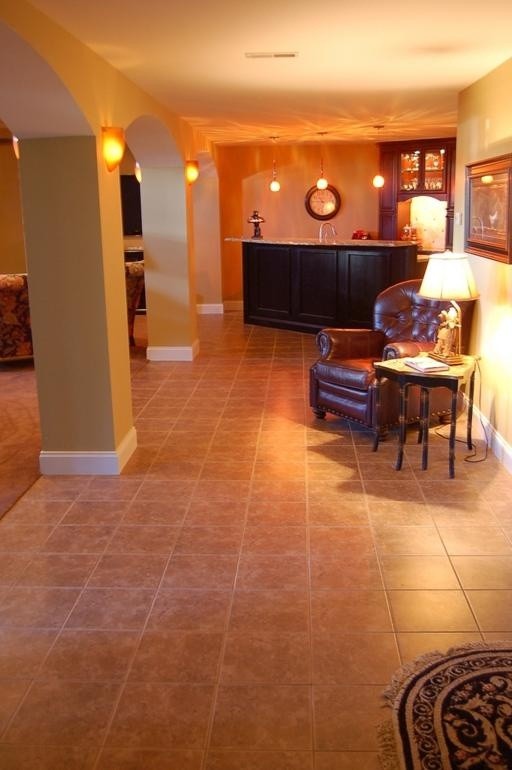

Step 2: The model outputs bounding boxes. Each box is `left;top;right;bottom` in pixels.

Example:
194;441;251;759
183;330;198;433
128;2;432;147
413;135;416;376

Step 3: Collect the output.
403;357;449;373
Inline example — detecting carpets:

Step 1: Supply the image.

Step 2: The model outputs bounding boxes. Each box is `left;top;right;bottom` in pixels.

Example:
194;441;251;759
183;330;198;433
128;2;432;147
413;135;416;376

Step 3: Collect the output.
0;343;149;528
374;641;510;765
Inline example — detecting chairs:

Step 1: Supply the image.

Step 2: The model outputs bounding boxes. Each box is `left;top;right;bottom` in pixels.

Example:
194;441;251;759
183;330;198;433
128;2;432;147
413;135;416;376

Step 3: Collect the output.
302;277;475;453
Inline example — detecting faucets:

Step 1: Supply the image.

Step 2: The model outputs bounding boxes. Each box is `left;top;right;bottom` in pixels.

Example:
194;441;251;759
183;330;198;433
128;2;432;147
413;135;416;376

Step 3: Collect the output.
320;221;338;242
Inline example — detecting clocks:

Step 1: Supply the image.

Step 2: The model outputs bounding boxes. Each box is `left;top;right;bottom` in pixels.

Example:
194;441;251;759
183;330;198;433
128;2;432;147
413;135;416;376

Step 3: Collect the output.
303;184;341;221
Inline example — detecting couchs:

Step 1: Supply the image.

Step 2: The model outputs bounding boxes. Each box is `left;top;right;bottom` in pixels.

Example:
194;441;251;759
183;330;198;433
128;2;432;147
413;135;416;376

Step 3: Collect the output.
1;257;146;362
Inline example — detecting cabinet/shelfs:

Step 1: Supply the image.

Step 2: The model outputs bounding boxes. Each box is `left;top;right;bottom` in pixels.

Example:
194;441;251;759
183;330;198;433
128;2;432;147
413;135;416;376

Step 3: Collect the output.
375;138;457;251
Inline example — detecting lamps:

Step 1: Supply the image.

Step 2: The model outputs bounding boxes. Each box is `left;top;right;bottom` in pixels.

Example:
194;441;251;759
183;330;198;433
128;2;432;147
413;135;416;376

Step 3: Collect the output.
313;132;330;190
101;124;126;173
268;136;281;192
414;247;478;367
371;123;386;190
186;159;200;187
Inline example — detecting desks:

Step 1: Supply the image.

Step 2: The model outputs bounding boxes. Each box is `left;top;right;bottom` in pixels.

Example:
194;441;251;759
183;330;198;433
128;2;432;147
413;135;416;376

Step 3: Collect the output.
370;351;479;481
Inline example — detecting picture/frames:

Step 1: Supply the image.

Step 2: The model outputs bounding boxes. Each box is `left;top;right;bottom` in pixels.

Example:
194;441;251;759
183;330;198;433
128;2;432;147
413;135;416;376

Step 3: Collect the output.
463;152;512;265
